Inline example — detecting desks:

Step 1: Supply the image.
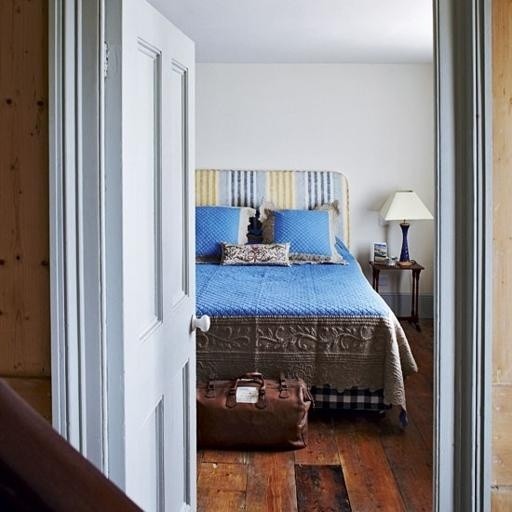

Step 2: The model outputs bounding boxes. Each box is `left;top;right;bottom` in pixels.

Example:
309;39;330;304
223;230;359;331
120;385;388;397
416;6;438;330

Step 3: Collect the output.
367;259;425;334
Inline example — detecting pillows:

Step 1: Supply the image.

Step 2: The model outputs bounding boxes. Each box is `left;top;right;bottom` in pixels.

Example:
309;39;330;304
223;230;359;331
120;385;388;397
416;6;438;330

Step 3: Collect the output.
198;199;351;270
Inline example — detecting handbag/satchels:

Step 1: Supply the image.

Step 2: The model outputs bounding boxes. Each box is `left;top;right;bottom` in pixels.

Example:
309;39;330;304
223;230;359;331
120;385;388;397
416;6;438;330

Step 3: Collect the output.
196;370;313;452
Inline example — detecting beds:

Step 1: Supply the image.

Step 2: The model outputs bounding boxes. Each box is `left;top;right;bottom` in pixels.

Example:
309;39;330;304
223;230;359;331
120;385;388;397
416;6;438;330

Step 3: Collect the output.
196;168;400;421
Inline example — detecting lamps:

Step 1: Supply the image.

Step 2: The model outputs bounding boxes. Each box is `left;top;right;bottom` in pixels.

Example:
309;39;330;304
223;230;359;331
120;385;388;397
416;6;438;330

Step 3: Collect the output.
379;190;435;266
